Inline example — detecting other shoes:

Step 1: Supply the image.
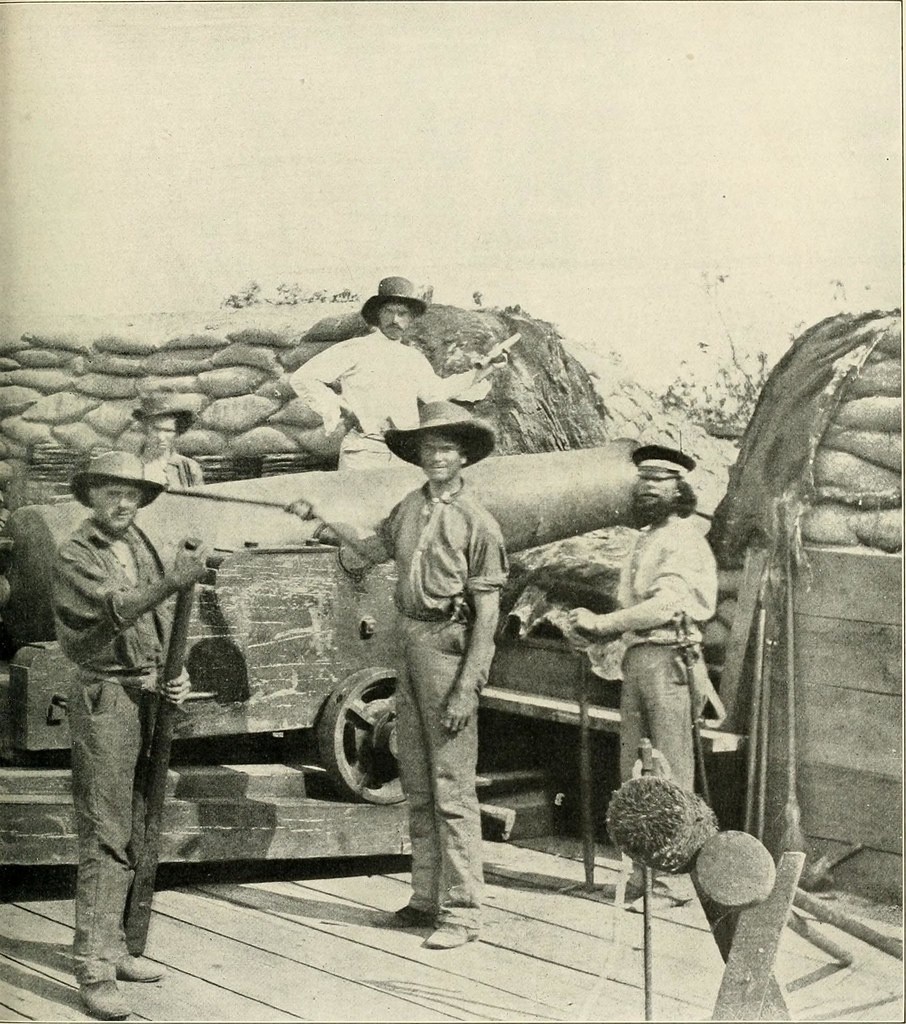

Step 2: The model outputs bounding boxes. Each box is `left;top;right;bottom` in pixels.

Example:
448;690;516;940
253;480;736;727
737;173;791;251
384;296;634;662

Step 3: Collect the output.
426;920;469;949
78;980;133;1019
369;906;437;929
631;890;682;913
116;952;166;982
603;882;644;899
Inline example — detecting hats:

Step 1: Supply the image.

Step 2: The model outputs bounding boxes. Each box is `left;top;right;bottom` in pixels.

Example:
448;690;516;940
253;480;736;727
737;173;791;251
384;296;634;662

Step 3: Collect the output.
632;445;697;479
71;451;164;510
384;401;496;470
360;277;427;328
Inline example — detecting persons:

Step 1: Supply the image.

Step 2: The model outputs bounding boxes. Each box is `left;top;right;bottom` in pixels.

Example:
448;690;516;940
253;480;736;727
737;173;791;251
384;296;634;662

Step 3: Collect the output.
291;279;517;475
48;447;218;1022
130;392;207;498
565;444;721;907
284;403;510;952
